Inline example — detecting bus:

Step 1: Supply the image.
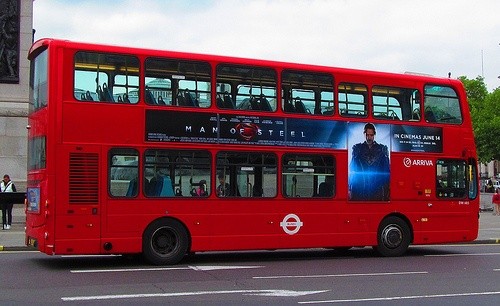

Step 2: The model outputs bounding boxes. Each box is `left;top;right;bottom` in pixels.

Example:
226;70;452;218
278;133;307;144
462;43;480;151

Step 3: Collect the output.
24;37;481;266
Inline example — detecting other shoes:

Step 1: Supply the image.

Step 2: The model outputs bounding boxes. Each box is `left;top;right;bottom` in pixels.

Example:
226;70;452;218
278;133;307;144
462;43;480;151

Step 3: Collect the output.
4;223;11;230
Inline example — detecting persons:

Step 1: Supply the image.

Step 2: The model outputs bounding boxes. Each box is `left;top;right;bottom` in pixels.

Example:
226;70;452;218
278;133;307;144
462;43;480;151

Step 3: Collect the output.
197;180;208;197
0;175;16;229
489;180;494;192
492;187;500;216
217;174;230;196
348;122;390;200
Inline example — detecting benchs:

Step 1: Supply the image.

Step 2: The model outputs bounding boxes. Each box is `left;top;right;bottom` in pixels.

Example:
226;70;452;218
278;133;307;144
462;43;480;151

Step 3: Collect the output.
82;83;437;123
128;175;174;197
